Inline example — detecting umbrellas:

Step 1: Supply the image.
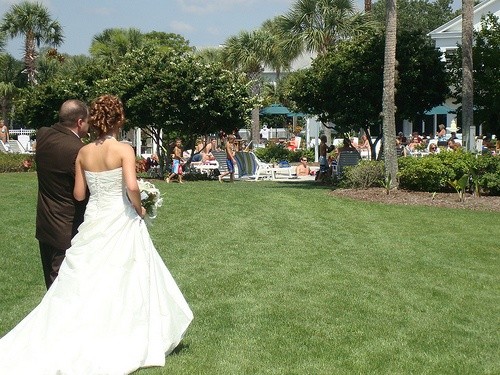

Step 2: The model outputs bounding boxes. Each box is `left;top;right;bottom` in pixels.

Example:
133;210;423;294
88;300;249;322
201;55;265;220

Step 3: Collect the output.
259;105;312;116
416;103;459;116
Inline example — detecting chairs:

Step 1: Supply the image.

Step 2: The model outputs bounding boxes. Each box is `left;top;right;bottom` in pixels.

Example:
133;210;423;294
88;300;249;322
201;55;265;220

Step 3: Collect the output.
191;151;275;180
330;150;403;178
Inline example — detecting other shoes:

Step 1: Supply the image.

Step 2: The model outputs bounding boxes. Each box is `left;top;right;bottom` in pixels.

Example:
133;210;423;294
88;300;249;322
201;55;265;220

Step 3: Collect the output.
218;175;222;183
165;177;170;184
178;175;184;184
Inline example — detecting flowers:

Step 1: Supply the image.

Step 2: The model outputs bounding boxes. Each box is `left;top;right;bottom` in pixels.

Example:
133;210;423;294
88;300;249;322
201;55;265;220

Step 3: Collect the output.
137;178;168;220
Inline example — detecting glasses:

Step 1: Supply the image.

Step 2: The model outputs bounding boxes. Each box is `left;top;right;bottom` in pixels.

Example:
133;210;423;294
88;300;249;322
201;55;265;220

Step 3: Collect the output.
302;160;306;161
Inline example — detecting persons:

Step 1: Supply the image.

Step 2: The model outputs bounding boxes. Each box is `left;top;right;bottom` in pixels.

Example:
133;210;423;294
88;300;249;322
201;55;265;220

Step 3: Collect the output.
35;99;91;291
317;135;333;183
218;135;238;183
285;134;298;152
296;156;315;178
0;119;9;152
166;137;185;184
476;134;500;157
337;138;357;152
195;129;228;160
396;124;460;157
0;95;194;375
357;134;370;148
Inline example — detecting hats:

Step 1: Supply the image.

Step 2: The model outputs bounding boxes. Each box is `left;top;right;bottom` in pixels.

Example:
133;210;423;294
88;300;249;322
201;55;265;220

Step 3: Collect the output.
263;125;267;130
